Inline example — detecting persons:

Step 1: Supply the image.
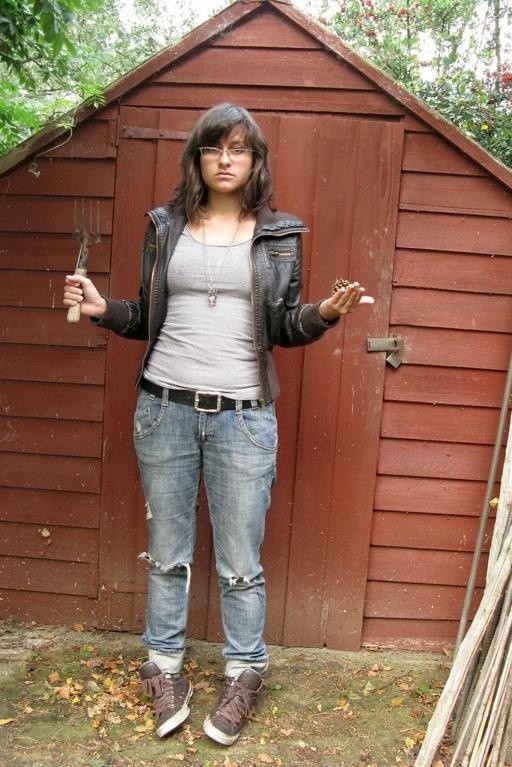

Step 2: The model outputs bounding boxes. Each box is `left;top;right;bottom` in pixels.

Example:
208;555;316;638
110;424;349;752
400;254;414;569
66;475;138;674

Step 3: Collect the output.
63;103;374;746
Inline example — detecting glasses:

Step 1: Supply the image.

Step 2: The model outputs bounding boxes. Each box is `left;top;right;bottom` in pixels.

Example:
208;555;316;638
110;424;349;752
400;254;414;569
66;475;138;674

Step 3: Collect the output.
197;144;256;162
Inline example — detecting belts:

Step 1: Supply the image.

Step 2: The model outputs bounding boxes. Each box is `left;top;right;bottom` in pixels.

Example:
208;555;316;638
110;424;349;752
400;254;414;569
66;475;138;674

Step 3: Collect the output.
138;372;262;412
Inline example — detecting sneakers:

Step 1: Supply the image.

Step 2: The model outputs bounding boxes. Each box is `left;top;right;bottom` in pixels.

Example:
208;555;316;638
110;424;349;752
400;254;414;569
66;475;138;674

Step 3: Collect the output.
204;667;264;746
140;660;194;737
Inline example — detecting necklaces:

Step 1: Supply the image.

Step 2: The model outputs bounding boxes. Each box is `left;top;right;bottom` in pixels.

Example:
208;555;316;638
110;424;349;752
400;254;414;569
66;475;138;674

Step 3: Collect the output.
202;217;242;307
